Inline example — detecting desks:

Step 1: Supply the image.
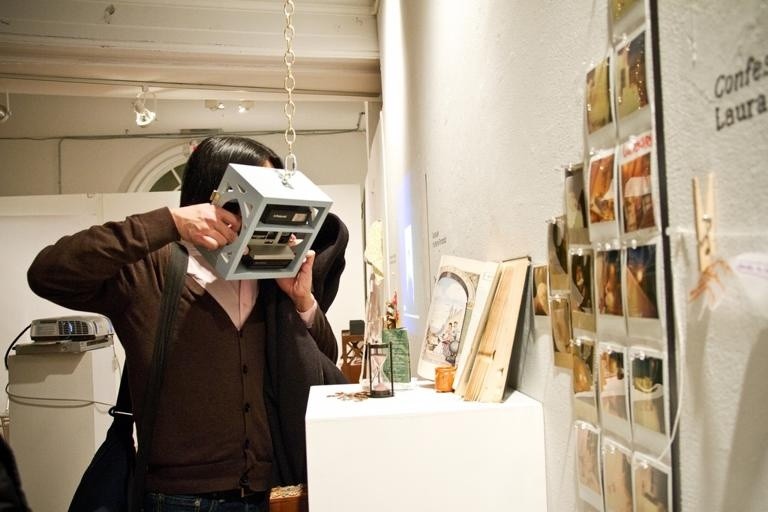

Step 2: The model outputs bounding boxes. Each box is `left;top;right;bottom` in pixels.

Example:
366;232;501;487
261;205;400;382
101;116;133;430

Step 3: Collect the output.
305;380;549;512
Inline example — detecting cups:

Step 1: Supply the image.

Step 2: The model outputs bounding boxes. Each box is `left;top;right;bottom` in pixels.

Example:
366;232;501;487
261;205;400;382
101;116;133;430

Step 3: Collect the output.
435;368;456;392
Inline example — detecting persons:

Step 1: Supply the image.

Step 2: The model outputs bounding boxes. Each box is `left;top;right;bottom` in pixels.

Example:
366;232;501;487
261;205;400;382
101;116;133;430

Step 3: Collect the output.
26;135;338;511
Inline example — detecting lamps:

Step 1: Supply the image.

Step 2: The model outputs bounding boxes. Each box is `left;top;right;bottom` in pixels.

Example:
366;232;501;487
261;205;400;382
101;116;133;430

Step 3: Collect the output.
132;92;160;127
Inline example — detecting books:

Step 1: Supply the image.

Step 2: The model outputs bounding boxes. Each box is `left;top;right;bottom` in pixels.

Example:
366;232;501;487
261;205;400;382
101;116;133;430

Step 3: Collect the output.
415;253;531;403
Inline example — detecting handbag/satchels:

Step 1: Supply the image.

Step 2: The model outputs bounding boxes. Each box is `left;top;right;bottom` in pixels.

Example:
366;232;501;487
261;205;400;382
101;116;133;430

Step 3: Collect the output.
62;240;189;508
